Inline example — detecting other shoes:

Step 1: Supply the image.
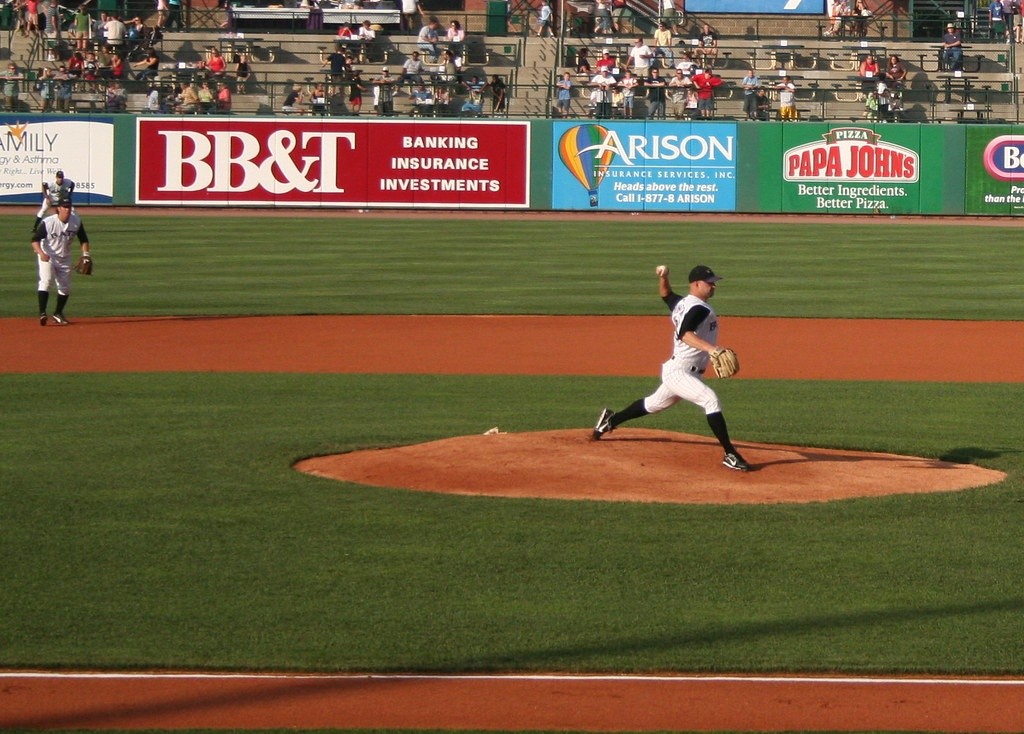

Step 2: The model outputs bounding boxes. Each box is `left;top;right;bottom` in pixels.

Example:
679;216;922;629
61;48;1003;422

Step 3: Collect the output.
31;228;38;232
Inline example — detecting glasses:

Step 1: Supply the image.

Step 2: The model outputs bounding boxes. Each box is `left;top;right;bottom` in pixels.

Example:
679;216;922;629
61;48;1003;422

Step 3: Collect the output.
677;73;682;75
8;68;17;70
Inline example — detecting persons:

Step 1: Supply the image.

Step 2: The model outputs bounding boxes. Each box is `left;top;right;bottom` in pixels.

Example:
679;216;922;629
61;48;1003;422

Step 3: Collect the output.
740;69;774;121
590;264;754;472
774;75;797;122
942;22;963;71
0;0;506;119
30;170;75;234
860;54;908;123
987;0;1024;44
823;0;873;37
30;197;93;326
535;0;556;38
556;0;724;120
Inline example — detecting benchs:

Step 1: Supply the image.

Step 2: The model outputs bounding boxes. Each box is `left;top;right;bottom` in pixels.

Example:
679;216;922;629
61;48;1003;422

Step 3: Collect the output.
0;46;992;124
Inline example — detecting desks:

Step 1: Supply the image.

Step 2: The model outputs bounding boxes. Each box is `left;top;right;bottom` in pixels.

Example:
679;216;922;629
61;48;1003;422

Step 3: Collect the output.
589;43;630;63
219;38;263;63
768;109;811;122
761;75;804;100
404;103;449;118
675;44;701;62
164;69;210;92
763;45;804;70
338;39;375;64
432;41;477;66
937;75;978;103
848;76;888;103
931;46;973;71
321;70;363;96
842;46;886;71
950;110;993;124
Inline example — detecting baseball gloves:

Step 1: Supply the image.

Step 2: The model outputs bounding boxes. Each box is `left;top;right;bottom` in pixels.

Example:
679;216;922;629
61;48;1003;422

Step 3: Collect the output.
709;345;739;378
70;253;91;274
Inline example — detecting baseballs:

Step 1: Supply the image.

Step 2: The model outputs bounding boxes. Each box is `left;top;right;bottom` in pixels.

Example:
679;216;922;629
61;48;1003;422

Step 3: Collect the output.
658;265;669;276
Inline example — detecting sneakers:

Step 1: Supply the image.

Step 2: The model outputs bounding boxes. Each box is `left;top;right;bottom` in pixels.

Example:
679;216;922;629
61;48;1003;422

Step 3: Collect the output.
39;313;47;326
592;409;618;441
723;451;750;471
52;314;69;324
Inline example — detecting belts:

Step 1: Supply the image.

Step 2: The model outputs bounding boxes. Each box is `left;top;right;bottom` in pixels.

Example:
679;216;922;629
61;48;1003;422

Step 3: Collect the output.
671;355;706;374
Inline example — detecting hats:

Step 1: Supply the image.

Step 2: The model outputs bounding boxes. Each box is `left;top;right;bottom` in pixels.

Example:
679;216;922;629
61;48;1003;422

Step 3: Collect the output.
946;23;954;28
59;198;72;207
689;265;723;283
601;66;608;72
382;67;389;71
602;49;610;54
57;171;63;178
